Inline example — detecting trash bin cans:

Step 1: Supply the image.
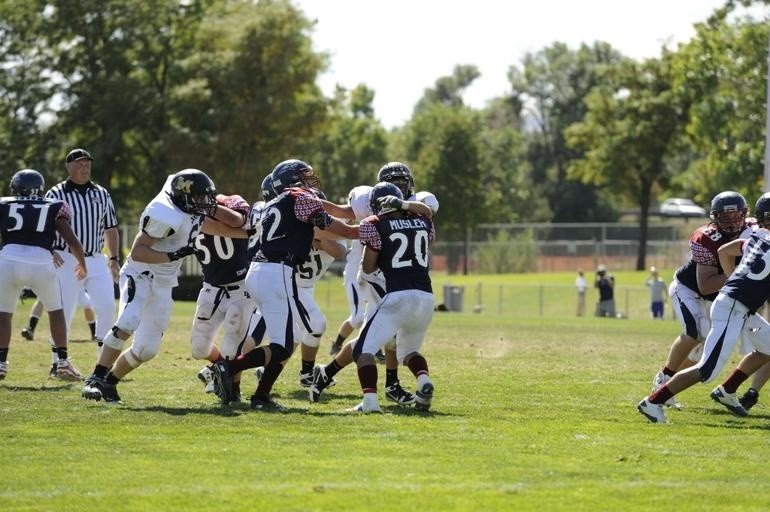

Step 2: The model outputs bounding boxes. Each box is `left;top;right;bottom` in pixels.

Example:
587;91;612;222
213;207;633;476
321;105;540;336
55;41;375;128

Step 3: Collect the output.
443;285;464;311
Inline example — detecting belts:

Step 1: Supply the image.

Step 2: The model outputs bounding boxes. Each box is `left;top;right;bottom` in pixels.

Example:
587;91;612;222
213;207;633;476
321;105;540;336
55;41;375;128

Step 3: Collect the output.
53;247;92;257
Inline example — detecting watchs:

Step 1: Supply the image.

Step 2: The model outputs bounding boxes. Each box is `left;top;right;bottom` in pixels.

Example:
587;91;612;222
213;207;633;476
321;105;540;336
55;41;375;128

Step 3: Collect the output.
108;255;120;261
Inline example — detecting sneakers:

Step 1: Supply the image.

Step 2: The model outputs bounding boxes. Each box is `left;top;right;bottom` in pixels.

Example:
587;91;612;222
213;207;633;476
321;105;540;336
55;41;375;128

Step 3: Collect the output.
50;363;57;377
414;378;434;411
0;361;9;379
385;379;416;406
329;341;342;355
256;366;265;382
651;371;674;406
56;356;81;379
21;326;34;341
103;376;125;406
375;349;385;360
710;384;748;417
232;389;240;402
245;392;288;413
205;379;214;394
345;402;383;414
637;396;666;422
300;369;335;389
82;374;104;401
738;388;759;410
309;364;335;403
214;356;233;405
197;365;214;385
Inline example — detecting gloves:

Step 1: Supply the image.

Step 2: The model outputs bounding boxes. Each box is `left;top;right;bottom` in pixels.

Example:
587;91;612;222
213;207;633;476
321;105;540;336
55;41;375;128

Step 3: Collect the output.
167;248;194;262
376;194;403;209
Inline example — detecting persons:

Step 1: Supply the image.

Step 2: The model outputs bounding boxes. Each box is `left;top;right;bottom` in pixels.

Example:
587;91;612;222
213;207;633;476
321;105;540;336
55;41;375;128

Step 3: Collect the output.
637;193;770;425
21;287;102;340
328;237;392;362
249;171;279;239
198;310;267;395
592;265;625;318
651;190;769;414
253;229;348;389
639;266;669;318
575;269;588;318
188;192;256;395
45;148;123;381
345;180;436;416
0;169;86;381
282;161;444;406
82;169;258;408
213;155;360;412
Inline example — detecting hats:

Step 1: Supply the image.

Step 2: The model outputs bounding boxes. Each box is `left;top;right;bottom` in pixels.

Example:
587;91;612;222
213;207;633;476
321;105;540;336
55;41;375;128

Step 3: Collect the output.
66;149;95;163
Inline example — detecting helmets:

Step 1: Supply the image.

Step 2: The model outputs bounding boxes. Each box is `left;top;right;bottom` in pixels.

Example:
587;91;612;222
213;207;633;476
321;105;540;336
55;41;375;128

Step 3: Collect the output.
707;190;748;238
9;168;46;197
261;173;277;203
165;168;218;218
368;182;403;215
754;191;770;232
376;161;415;200
270;159;321;195
